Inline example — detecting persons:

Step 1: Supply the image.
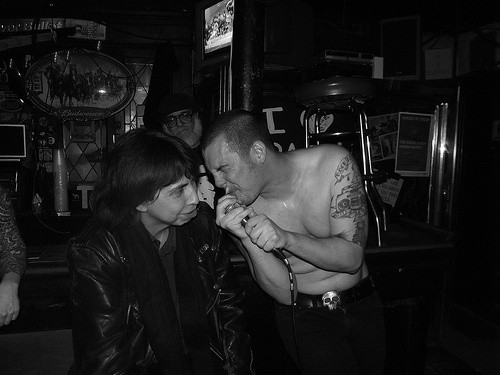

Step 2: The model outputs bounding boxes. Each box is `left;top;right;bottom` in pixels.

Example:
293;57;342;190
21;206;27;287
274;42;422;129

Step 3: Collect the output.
199;109;386;375
0;185;28;327
160;93;227;218
68;127;256;375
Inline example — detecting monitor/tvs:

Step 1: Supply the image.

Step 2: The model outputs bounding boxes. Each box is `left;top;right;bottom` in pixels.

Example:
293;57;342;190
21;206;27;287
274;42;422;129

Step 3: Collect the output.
201;0;234;60
0;124;26;158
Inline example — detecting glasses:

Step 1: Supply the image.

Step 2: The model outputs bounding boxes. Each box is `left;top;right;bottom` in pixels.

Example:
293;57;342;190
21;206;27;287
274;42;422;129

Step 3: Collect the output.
165;112;195;129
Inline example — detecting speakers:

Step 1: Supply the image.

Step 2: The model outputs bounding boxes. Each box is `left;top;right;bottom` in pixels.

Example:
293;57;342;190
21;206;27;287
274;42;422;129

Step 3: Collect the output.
381;15;424;80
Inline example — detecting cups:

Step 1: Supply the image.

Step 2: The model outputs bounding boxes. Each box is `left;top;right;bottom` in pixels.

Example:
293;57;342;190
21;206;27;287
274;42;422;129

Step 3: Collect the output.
52;148;70;212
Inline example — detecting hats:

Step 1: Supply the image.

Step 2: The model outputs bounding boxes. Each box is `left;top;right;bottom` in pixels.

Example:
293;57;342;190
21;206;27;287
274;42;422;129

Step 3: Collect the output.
161;94;203;117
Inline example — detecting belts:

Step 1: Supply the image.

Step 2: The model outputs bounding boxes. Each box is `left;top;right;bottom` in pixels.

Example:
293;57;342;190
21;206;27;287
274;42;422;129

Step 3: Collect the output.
295;276;374;309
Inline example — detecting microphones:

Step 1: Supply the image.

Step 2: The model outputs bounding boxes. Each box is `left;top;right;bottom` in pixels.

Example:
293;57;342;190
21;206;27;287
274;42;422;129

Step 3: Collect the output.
225;204;288;263
